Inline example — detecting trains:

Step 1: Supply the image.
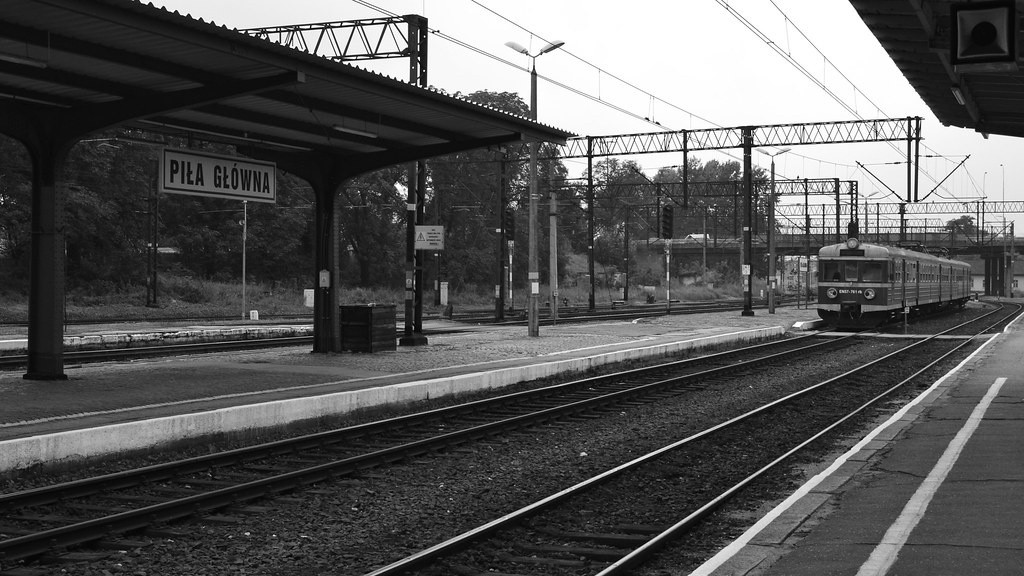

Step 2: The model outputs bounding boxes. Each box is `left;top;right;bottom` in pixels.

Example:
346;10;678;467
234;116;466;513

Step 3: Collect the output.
813;221;973;331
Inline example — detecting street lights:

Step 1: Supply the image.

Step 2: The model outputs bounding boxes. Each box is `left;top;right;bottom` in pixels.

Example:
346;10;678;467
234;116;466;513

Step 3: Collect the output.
504;36;566;338
754;147;791;314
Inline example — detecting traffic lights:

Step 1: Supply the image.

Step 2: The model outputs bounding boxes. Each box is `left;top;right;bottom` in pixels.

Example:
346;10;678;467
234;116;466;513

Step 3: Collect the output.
661;205;674;239
504;207;515;242
